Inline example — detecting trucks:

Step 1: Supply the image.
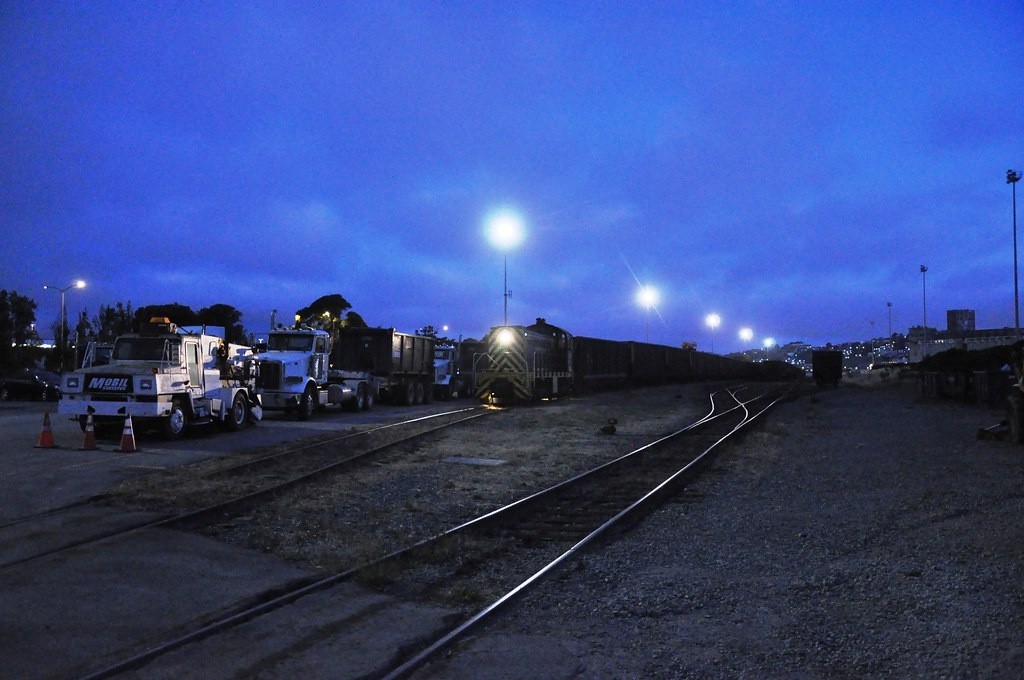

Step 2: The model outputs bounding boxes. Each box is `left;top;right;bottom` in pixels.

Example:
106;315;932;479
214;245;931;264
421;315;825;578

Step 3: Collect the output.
433;344;460;386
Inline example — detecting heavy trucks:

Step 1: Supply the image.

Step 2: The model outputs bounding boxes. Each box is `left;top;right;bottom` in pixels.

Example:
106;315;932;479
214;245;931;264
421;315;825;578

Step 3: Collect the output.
255;313;436;420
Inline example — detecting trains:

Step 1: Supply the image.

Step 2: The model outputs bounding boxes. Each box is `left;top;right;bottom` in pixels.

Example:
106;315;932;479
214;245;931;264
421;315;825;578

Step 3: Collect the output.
472;317;755;401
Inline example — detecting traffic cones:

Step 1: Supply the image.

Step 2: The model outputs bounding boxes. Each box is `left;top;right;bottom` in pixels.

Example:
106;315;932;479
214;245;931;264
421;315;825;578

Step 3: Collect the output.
77;413;100;451
114;412;141;452
34;408;59;450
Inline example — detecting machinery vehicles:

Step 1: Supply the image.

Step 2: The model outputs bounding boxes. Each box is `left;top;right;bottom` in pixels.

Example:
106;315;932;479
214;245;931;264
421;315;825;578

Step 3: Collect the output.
58;316;261;441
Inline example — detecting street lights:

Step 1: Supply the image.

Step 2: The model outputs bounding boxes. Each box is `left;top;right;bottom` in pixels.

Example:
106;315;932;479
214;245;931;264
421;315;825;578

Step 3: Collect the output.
485;209;524;325
884;301;895;343
704;312;723;352
636;283;657;344
1005;167;1024;329
42;280;86;359
919;264;930;357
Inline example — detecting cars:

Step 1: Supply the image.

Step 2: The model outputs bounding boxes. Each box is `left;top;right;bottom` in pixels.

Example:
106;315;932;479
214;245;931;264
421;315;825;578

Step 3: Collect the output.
0;367;61;401
